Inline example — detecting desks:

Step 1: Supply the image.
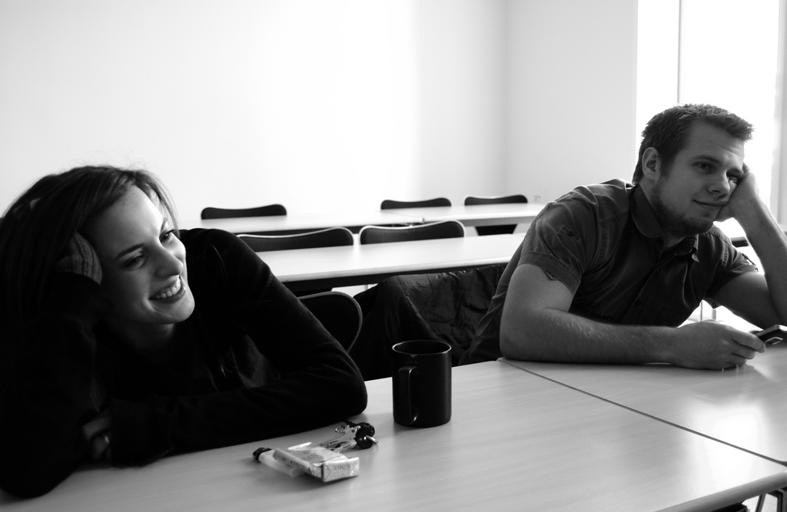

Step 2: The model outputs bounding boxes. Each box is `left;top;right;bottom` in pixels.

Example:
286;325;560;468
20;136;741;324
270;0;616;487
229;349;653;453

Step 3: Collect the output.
180;202;548;240
2;319;787;510
254;232;531;289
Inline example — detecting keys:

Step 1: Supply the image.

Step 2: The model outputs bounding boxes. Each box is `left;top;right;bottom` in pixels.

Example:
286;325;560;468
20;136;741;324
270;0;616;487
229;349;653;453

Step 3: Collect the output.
321;417;376;453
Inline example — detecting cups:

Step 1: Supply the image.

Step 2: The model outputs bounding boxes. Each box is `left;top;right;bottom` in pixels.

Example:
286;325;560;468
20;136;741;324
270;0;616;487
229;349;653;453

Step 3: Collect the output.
391;338;452;428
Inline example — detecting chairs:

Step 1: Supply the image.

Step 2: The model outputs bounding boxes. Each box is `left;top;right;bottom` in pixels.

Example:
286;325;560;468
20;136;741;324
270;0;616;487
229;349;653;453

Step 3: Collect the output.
202;204;286;222
237;226;355;253
464;195;529;235
360;218;466;242
294;289;364;356
382;196;451;213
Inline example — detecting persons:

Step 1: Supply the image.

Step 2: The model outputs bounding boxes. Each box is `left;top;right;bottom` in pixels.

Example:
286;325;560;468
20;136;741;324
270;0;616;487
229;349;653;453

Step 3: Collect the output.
455;103;785;369
0;167;365;498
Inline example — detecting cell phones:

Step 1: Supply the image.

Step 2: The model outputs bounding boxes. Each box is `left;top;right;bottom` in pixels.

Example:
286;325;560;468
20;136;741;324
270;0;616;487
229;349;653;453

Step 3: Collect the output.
753;322;787;347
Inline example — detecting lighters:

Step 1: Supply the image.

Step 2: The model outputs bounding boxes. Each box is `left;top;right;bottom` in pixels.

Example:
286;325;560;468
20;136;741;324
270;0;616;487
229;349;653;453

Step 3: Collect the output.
251;445;305;478
